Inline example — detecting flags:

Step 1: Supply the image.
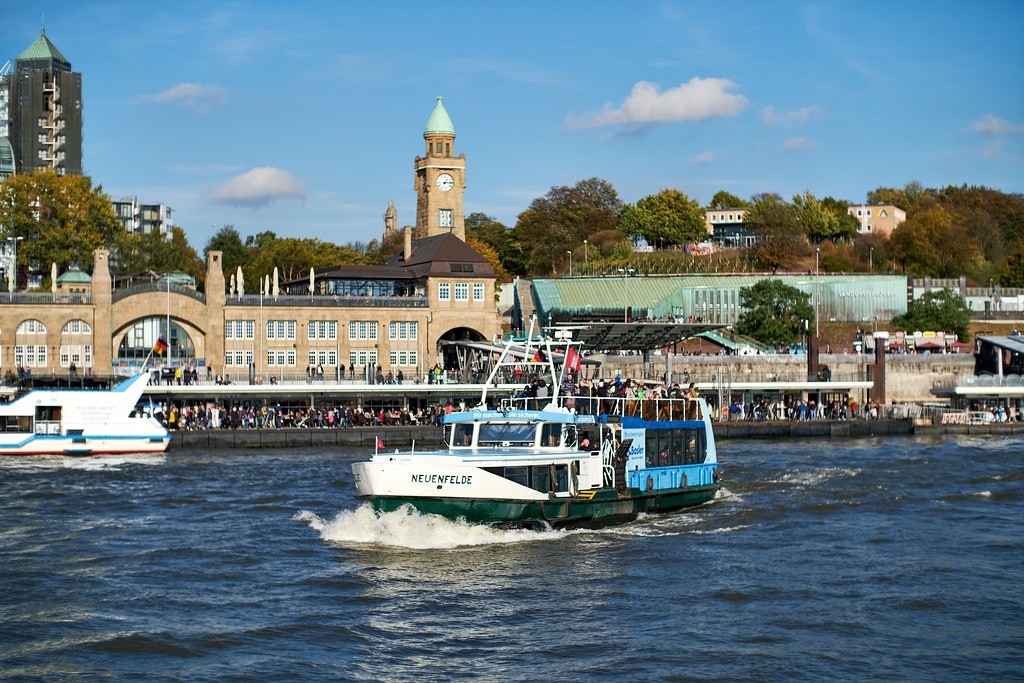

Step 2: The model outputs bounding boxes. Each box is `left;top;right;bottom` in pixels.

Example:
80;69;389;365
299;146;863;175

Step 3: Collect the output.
153;338;168;356
531;348;549;363
566;347;583;372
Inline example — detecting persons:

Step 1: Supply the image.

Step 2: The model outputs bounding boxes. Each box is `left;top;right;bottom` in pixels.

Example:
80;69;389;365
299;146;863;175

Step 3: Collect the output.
216;374;231;385
154;366;199;386
336;362;403;385
25;365;31;377
270;374;276;385
581;439;593;451
628;314;703;323
975;402;1010;423
519;374;700;420
428;363;444;384
306;362;324;380
16;364;25;379
730;398;881;423
207;363;211;381
154;401;462;432
384;201;397;236
70;363;76;374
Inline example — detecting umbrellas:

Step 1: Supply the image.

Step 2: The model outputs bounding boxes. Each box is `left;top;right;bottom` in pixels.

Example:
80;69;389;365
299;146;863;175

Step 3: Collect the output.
890;340;974;354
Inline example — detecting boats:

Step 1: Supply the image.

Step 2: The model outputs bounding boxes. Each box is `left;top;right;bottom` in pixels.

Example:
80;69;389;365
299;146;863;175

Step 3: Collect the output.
0;371;172;456
349;313;727;532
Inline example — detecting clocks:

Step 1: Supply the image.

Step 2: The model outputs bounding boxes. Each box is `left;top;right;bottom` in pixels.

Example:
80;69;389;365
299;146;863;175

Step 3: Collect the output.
436;174;455;192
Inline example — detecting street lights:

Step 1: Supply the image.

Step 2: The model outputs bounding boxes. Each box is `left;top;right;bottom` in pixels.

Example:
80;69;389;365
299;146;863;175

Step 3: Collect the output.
7;236;25;295
990;278;993;291
584;240;587;263
567;250;571;278
870;246;875;275
617;268;635;323
815;248;820;340
